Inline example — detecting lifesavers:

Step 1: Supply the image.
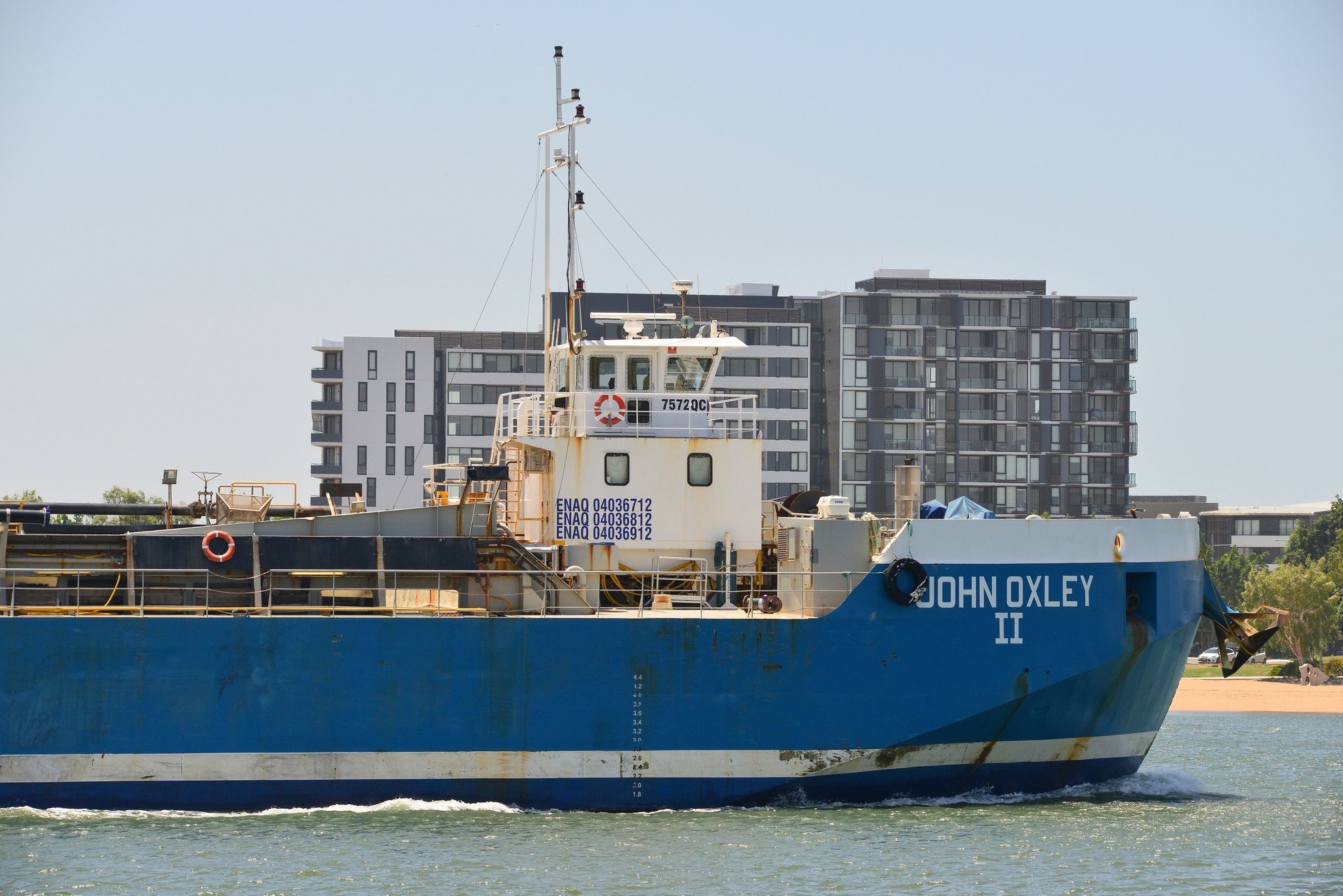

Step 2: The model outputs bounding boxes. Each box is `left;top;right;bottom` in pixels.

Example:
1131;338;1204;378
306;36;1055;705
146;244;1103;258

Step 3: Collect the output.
594;395;626;426
202;530;235;562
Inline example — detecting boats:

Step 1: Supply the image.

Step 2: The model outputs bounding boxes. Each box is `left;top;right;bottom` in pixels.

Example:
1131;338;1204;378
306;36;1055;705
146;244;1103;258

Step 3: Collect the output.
0;45;1277;813
